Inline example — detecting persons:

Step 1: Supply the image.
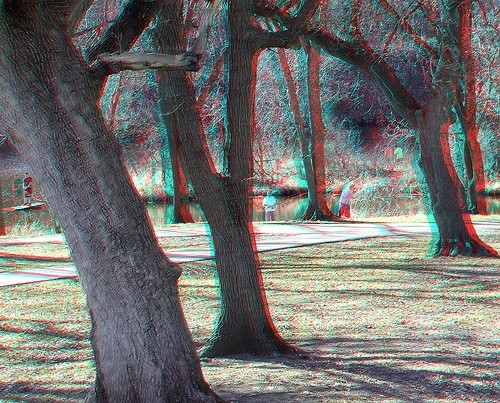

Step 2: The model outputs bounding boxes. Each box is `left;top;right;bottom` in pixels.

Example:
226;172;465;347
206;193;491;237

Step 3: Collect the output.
330;187;342;217
253;192;265;221
23;172;33;206
339;184;356;219
394;144;403;162
11;173;24;206
263;191;276;221
383;144;394;159
